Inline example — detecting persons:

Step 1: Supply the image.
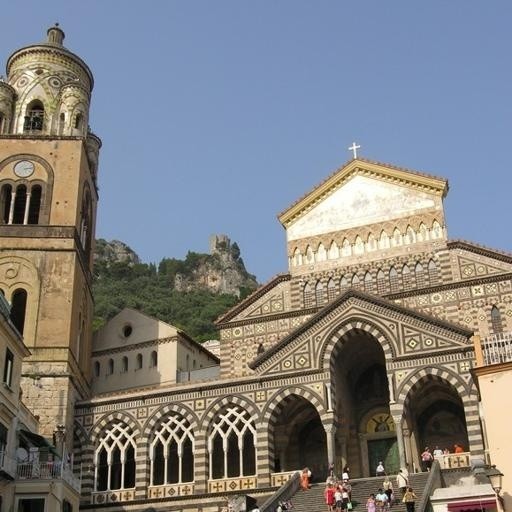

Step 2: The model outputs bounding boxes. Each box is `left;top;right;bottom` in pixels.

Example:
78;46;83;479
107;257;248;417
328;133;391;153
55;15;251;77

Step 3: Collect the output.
375;461;387;476
432;445;444;457
365;463;419;512
46;453;61;478
276;497;296;512
442;448;451;455
18;451;29;479
251;505;261;512
322;458;355;512
420;446;434;472
453;444;465;453
299;464;310;491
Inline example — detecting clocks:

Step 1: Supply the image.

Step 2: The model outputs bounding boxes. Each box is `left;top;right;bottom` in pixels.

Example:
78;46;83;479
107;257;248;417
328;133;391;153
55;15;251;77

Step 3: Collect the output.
13;160;35;178
